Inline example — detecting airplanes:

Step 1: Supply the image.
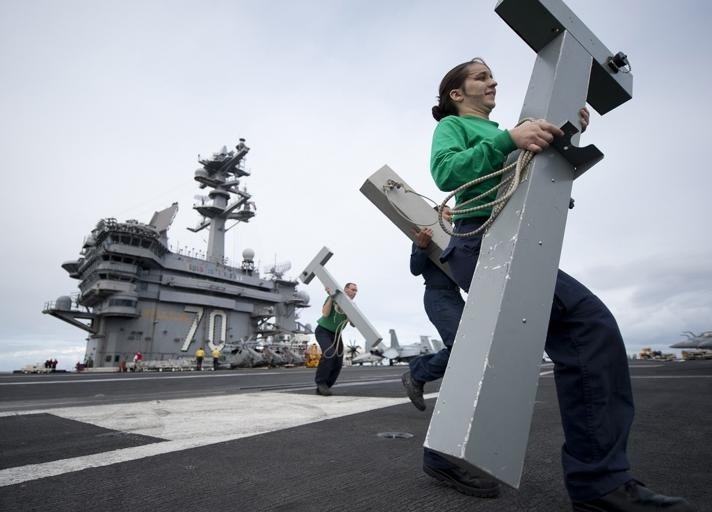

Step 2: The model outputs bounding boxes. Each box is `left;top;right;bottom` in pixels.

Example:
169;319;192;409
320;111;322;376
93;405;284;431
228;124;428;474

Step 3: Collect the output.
345;328;446;367
669;331;712;350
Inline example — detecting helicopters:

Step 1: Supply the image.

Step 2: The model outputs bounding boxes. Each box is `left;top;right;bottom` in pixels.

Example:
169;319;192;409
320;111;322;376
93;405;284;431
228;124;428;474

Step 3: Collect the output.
217;335;310;369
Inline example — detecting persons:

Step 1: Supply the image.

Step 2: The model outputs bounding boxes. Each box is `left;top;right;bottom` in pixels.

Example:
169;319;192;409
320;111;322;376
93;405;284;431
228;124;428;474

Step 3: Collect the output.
133;352;144;372
196;348;204;370
314;282;358;395
212;347;220;371
422;57;689;511
400;205;465;411
45;359;57;373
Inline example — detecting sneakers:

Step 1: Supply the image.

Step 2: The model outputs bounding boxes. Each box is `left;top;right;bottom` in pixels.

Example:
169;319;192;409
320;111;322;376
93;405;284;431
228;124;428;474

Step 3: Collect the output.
572;478;698;511
422;448;501;498
402;371;426;411
317;382;332;395
